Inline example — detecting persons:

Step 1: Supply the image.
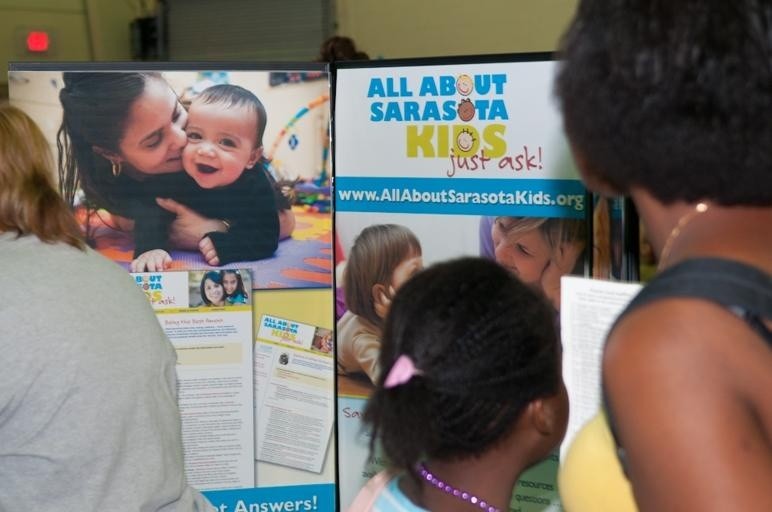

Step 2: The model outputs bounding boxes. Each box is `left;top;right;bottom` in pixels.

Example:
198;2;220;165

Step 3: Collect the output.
199;272;225;306
316;36;365;63
128;83;295;271
480;216;583;322
555;0;772;512
57;73;296;246
220;270;246;304
0;103;218;512
344;254;570;512
336;223;421;387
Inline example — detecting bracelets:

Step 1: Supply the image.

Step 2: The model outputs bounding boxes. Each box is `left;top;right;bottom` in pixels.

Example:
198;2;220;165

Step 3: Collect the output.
220;218;232;228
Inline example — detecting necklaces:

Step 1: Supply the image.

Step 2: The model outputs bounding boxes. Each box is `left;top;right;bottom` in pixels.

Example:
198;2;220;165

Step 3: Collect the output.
657;202;708;270
416;463;501;512
